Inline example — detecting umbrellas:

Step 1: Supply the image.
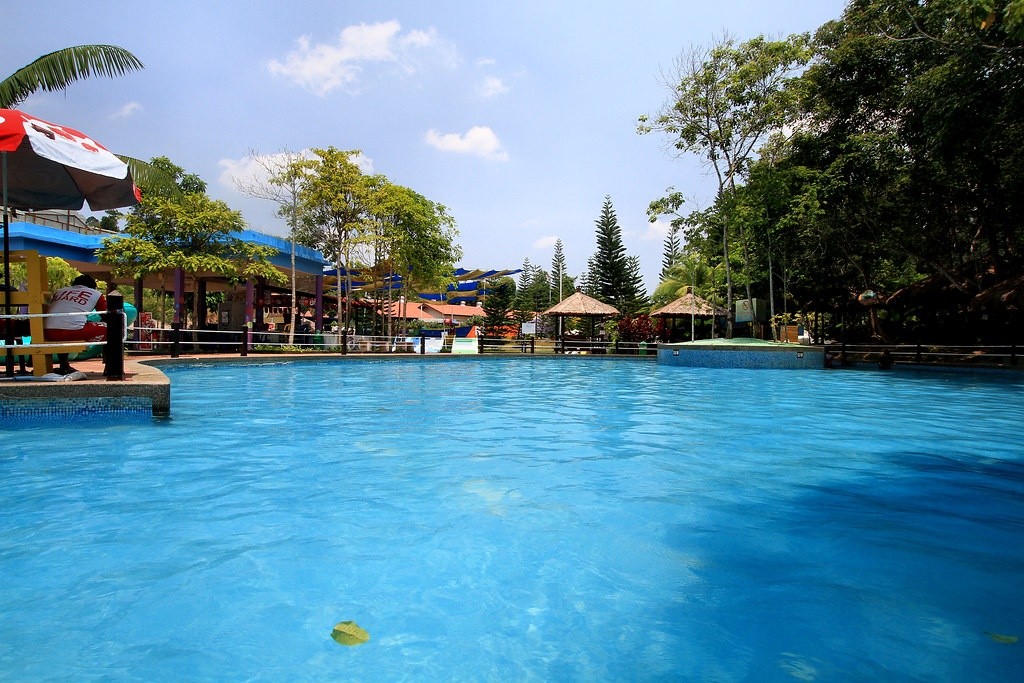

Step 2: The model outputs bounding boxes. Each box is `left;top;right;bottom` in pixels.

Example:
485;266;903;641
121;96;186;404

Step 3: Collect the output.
542;292;620;353
0;108;142;377
649;293;728;343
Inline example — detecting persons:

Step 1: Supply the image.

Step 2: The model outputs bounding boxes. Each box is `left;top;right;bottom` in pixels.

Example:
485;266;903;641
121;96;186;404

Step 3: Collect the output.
44;274;107;376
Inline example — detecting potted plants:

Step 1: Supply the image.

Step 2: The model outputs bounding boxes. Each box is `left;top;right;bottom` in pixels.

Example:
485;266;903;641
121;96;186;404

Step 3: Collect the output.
446;327;455;343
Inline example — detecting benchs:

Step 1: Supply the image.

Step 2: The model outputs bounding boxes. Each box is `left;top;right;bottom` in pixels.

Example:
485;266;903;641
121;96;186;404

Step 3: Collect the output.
0;248;86;376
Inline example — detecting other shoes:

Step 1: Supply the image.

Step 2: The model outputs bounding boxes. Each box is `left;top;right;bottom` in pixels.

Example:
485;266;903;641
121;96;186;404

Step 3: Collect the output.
102;365;125;376
60;363;77;374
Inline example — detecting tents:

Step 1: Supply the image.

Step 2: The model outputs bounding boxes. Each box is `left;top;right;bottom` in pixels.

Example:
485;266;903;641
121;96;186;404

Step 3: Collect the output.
0;220;525;348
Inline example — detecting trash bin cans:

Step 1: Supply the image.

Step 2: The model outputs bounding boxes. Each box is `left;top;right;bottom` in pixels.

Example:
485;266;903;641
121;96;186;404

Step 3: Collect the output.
639;341;647;355
414;329;419;336
313;336;324;350
798;325;804;335
